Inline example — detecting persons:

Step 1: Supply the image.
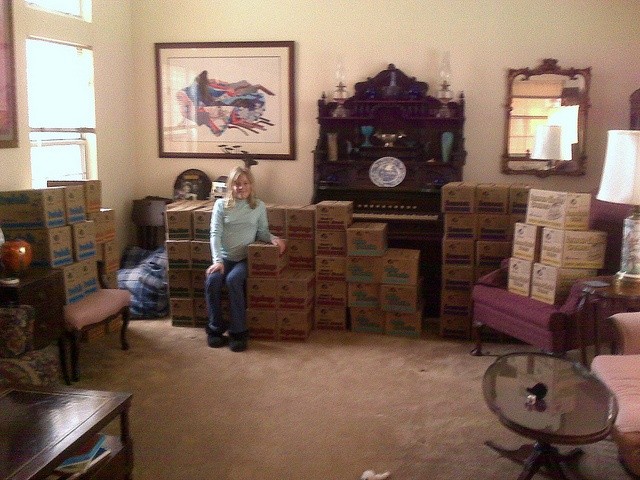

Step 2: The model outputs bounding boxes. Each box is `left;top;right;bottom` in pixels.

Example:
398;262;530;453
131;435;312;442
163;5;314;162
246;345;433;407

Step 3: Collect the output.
205;165;286;352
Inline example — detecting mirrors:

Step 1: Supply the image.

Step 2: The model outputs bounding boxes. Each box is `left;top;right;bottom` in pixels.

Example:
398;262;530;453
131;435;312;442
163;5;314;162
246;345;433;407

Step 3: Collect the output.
501;58;591;177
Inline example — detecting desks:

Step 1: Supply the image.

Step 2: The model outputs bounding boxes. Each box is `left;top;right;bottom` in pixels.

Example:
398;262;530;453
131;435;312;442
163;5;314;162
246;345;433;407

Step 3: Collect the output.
0;382;134;479
0;269;72;384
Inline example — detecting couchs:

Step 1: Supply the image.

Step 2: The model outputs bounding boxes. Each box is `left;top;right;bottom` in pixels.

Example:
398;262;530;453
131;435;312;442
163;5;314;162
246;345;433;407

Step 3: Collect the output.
589;312;640;478
471;190;640;357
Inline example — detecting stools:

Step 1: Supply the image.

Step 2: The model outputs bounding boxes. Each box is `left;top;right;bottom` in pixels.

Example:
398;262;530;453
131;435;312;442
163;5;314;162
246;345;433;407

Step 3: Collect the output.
63;289;130;381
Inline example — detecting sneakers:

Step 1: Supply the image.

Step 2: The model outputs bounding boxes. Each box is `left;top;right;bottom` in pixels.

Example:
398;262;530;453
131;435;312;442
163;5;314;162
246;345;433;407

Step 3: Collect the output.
228;332;247;351
207;328;226;348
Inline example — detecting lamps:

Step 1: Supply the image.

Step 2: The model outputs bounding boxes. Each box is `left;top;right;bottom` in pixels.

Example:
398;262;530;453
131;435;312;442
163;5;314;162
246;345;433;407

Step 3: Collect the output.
596;130;640;297
530;126;561;161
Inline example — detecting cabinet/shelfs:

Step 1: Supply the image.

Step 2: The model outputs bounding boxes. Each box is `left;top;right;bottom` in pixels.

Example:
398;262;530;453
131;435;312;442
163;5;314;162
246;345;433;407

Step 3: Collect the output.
313;64;466;192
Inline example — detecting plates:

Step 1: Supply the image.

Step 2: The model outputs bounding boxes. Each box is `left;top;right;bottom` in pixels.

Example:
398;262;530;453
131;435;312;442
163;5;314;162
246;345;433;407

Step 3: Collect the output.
368;156;407;188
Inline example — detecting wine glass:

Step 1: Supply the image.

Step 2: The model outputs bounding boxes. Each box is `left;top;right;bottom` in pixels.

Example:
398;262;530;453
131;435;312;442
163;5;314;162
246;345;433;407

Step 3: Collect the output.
361;126;374;147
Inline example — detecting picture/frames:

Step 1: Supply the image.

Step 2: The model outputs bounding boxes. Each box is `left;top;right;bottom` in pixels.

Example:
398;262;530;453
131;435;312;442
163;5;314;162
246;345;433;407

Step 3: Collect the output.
154;41;295;160
1;0;20;148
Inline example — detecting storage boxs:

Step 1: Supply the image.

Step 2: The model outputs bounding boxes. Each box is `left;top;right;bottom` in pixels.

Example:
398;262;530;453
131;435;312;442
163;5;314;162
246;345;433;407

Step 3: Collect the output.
81;324;106;343
440;182;475;340
526;187;592;231
512;221;540;263
476;215;510;241
1;179;119;269
508;259;533;298
107;315;122;334
46;269;118;305
164;199;231;329
476;241;511;285
509;183;530;221
530;263;597;307
246;201;422;342
540;228;608;270
475;182;509;215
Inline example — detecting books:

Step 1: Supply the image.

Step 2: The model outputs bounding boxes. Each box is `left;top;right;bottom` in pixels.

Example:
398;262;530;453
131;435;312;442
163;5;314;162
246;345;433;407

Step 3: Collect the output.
55;433;111;480
